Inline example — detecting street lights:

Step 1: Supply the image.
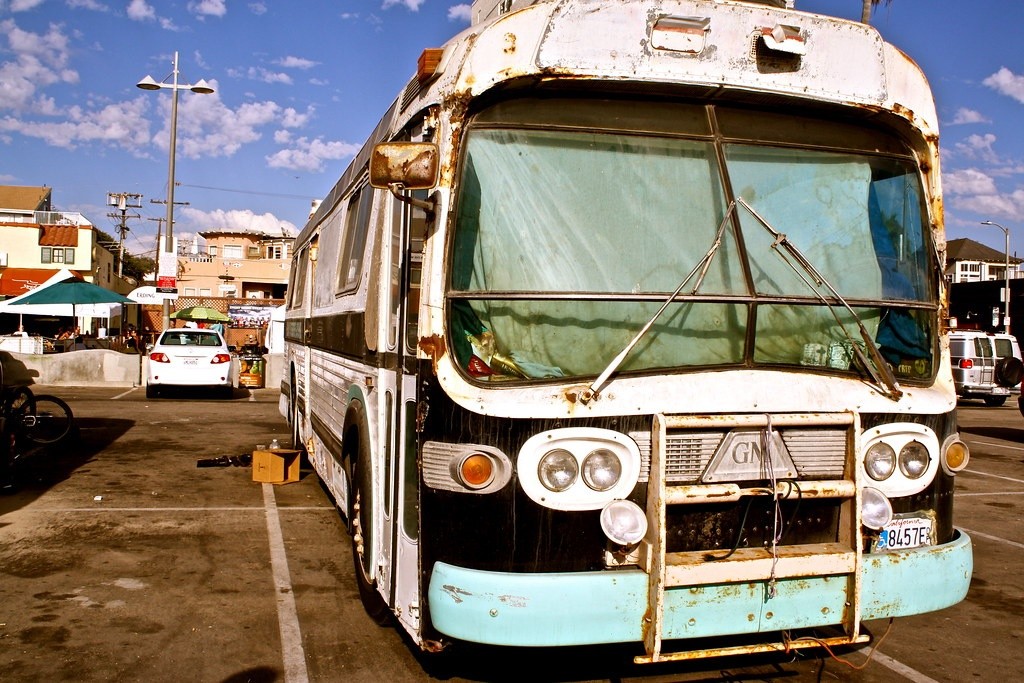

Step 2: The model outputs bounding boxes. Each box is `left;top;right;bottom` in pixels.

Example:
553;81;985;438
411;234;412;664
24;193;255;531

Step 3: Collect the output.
981;220;1010;335
137;51;214;331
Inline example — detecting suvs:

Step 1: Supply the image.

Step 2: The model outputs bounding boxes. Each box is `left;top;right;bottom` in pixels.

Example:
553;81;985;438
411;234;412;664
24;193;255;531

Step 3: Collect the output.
947;329;1024;406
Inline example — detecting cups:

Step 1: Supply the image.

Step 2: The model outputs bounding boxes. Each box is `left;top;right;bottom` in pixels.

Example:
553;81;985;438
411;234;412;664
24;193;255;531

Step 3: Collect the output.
256;444;266;450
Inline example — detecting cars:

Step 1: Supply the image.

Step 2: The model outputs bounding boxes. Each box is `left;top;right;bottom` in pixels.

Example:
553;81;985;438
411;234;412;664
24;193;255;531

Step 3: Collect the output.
146;328;236;399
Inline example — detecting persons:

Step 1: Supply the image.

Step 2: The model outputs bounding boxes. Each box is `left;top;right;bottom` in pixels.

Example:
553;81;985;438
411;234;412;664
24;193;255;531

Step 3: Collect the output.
53;320;224;354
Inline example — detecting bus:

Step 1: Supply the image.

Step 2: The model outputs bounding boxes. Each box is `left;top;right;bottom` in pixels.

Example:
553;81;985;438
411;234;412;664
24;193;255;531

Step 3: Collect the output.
280;0;973;665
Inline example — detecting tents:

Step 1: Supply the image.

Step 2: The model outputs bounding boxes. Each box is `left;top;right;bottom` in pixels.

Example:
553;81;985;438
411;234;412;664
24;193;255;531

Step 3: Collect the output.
0;269;122;339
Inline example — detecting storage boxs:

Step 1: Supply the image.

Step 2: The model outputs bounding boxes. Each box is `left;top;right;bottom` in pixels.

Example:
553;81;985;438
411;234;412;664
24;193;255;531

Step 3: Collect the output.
252;449;302;485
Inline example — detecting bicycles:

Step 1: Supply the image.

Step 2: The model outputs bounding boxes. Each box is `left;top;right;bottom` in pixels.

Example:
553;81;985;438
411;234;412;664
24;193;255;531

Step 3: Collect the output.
0;387;73;494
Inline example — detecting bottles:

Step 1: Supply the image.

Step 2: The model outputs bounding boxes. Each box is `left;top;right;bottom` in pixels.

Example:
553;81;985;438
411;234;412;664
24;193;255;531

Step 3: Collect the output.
244;334;254;344
228;315;265;329
268;439;281;449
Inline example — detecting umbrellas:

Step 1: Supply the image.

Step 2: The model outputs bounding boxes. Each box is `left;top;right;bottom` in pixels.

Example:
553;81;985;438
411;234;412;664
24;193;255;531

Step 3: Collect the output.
170;306;235;325
7;275;137;351
